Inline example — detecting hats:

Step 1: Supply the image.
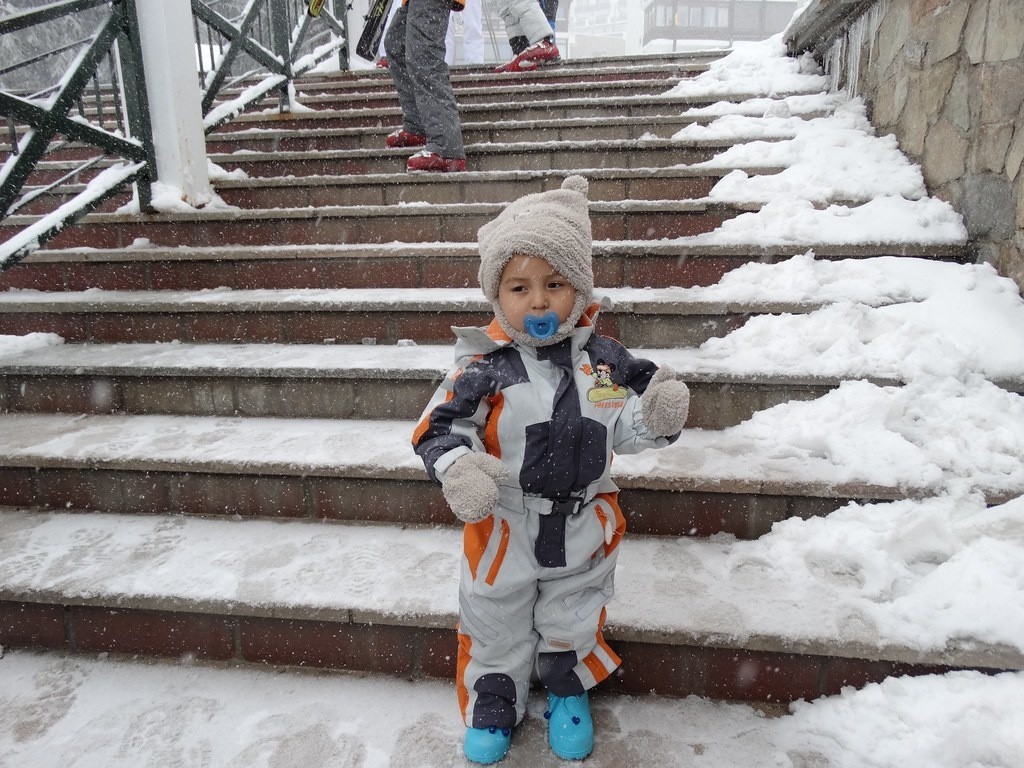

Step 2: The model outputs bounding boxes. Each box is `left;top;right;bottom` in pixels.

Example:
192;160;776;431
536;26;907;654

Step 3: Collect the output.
476;175;594;349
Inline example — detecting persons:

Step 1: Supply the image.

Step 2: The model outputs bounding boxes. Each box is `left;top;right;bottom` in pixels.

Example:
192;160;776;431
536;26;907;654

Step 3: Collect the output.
493;0;561;74
412;173;691;764
384;0;467;173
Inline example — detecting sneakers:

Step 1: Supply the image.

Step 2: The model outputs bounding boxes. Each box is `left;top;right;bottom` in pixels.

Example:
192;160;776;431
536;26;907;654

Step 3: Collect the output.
518;37;561;62
376;56;389;67
493;52;537;72
464;705;527;764
544;687;593;760
406;145;466;172
386;129;426;147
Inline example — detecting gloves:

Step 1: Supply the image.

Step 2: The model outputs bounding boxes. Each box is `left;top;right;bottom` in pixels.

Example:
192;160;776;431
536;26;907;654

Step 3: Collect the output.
440;450;511;525
641;366;690;440
